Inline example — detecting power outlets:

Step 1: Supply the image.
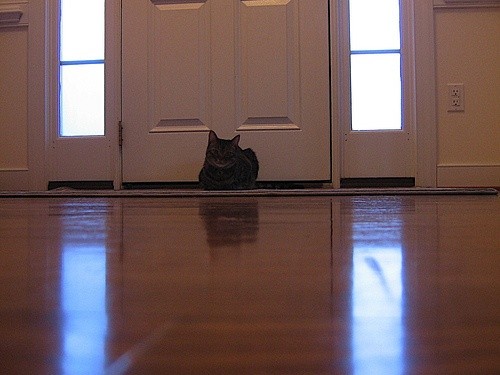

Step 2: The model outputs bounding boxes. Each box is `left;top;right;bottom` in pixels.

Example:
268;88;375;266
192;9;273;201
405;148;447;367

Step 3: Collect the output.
446;83;465;112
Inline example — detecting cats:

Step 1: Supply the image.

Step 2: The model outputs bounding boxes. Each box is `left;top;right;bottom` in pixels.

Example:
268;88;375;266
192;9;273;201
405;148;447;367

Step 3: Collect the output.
198;130;259;190
